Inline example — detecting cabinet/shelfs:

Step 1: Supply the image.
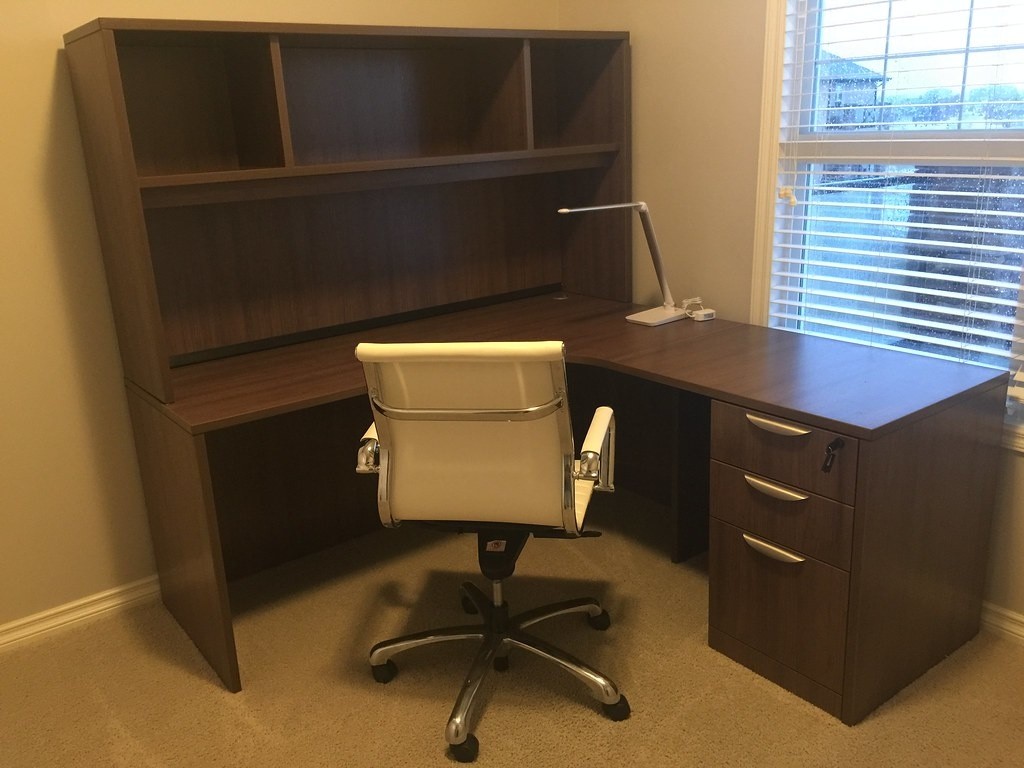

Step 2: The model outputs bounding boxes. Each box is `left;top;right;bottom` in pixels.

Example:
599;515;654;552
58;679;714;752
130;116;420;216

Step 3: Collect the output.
63;16;632;402
704;396;858;730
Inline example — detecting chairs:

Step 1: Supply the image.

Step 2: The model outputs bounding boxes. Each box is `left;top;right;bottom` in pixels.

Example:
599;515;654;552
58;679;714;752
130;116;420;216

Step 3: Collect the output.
353;338;633;762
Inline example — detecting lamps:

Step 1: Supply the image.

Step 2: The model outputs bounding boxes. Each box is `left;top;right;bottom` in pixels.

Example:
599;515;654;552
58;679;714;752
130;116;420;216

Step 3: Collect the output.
556;200;717;328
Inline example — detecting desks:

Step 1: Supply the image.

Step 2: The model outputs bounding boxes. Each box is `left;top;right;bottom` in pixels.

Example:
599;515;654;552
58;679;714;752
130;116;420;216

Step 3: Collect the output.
119;293;1015;726
